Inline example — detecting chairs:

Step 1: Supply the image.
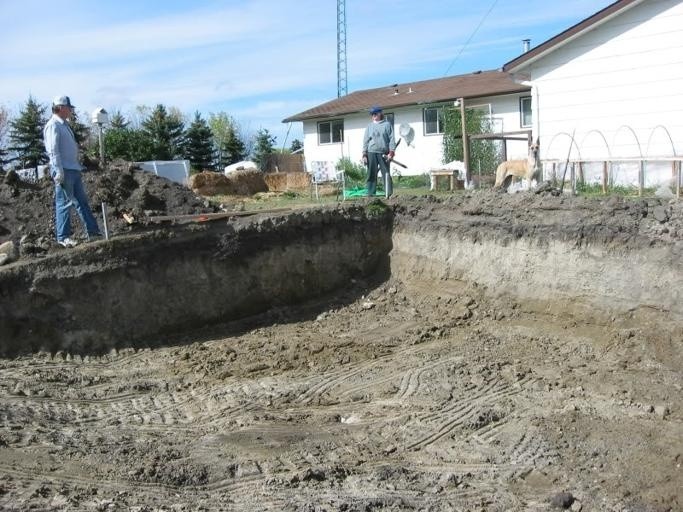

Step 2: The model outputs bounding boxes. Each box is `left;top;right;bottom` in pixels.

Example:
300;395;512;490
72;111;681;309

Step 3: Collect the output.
309;160;346;203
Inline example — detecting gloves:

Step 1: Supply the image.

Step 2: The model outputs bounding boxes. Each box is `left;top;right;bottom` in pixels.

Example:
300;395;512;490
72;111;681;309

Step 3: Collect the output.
388;151;394;161
54;173;64;185
363;154;368;166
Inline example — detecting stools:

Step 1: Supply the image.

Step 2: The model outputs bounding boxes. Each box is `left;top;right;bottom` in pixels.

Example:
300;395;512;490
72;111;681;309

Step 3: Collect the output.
430;170;459;192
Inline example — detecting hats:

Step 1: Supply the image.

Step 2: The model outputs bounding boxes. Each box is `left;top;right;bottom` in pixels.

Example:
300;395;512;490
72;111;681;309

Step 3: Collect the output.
54;96;75;108
368;106;381;114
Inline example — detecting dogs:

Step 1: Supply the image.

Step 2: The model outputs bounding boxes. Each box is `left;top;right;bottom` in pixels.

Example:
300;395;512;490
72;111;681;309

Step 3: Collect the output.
493;135;543;191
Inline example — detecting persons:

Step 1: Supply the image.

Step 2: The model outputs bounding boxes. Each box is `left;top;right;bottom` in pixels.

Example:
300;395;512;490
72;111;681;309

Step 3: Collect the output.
41;95;102;248
362;105;395;198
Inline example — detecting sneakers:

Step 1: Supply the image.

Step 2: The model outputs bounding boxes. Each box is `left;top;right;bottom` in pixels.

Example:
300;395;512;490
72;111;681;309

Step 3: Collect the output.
88;231;102;241
58;238;77;248
